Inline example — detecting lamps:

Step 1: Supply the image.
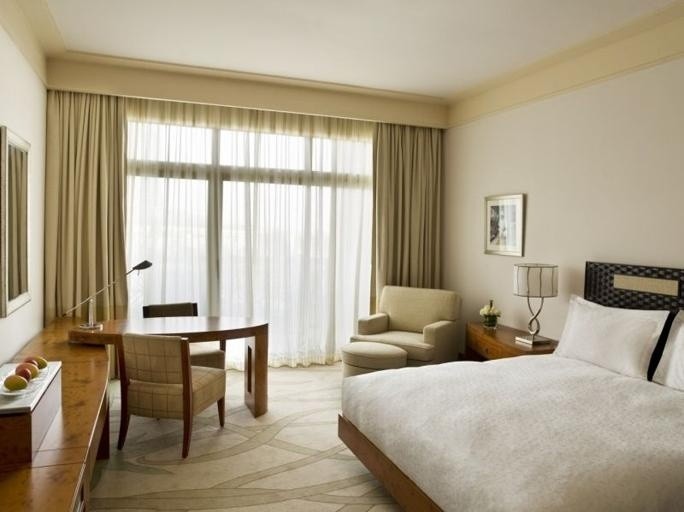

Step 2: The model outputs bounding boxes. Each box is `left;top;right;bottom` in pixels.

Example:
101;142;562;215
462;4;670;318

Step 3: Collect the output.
63;259;152;316
513;263;558;345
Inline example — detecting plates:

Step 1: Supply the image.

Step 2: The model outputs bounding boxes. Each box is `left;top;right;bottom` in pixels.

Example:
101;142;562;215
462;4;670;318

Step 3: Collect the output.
0;361;50;396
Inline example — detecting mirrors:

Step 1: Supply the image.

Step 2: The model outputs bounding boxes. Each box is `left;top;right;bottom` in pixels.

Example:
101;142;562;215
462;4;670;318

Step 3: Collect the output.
0;126;32;318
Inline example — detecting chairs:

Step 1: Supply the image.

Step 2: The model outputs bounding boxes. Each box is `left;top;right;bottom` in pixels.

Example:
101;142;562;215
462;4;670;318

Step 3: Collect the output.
350;285;461;367
116;332;226;458
143;303;226;369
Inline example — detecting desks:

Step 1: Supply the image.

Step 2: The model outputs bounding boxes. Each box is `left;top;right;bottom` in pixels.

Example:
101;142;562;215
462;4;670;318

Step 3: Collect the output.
466;322;559;360
0;317;111;512
68;316;268;418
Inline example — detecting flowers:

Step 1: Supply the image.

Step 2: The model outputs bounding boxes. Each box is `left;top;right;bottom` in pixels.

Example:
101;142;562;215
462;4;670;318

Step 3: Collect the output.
480;305;501;317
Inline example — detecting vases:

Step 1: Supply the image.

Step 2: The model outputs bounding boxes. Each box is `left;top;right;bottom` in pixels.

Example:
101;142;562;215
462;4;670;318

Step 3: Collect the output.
484;314;497;327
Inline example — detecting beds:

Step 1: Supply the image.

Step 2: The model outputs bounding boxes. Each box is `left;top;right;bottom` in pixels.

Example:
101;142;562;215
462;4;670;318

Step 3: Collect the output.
339;261;684;512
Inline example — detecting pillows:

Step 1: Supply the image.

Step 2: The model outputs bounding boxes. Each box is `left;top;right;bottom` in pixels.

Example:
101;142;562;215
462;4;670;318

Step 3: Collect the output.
553;294;670;381
652;310;684;391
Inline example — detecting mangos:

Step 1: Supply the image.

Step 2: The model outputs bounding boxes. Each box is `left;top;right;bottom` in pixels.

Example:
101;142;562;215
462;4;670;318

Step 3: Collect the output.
4;376;27;391
24;355;47;369
16;363;39;378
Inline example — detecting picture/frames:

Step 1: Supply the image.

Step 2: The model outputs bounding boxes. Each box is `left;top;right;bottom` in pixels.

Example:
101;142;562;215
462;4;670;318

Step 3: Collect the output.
484;194;523;257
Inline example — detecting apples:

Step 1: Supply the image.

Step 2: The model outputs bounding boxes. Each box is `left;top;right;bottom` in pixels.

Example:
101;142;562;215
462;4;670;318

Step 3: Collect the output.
19;368;31;381
26;359;39;367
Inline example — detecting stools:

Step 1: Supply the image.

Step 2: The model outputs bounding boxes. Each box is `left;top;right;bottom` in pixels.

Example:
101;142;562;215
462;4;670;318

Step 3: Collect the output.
341;342;408;378
